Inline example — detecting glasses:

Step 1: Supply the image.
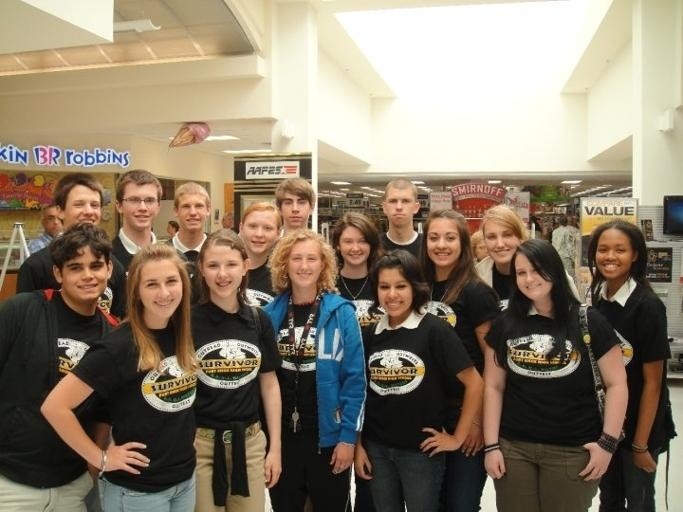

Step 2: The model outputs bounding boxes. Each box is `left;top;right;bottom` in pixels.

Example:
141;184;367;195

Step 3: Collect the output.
121;195;159;205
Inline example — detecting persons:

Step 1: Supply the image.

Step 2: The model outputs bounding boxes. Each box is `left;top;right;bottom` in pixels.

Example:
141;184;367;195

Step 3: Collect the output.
482;236;632;512
1;224;116;510
580;219;679;512
37;243;198;510
257;231;357;511
179;226;284;510
17;168;597;333
344;253;481;510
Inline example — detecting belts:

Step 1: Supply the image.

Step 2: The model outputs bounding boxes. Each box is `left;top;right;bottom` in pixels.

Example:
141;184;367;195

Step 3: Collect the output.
195;421;262;443
